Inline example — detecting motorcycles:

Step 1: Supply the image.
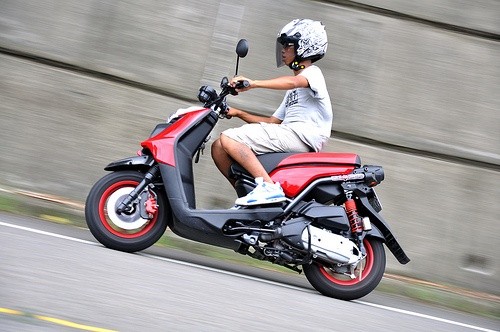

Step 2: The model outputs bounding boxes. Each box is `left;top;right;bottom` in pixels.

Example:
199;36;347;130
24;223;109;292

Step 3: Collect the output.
85;39;411;301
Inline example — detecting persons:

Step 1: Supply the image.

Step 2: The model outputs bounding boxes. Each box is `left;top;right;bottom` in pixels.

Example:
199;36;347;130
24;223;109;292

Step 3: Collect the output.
210;16;336;212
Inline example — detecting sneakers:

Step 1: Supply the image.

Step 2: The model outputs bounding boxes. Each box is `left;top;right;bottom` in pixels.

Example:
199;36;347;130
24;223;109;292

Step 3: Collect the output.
235;178;286;205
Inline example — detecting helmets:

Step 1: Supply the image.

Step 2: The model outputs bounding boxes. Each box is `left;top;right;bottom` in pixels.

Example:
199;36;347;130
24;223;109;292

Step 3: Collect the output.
274;18;328;67
